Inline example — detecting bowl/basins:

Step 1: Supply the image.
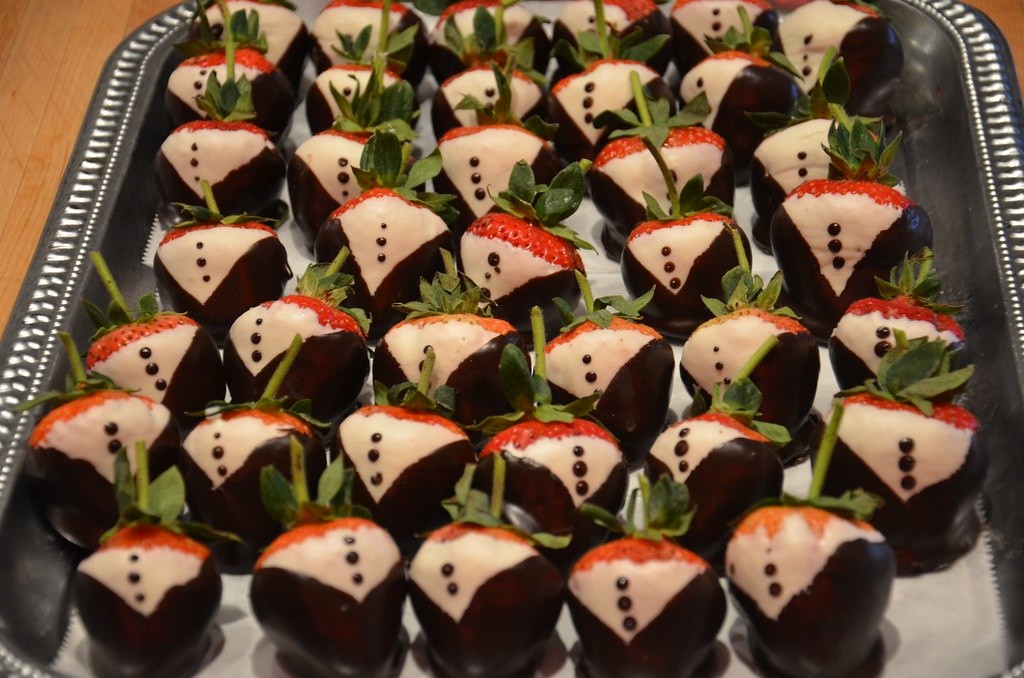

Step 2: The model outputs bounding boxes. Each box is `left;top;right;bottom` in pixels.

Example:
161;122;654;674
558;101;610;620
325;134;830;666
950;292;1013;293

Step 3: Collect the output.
0;0;1024;678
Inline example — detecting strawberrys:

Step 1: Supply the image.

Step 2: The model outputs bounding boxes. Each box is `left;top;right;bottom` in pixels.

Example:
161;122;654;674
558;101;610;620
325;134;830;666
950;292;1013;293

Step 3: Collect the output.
28;0;988;678
